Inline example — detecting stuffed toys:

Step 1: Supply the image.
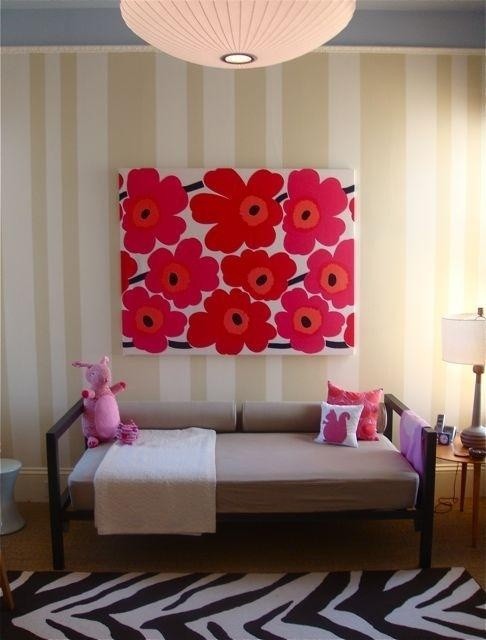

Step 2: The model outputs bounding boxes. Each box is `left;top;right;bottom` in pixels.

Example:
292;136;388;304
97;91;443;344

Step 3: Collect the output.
114;419;138;447
71;355;128;446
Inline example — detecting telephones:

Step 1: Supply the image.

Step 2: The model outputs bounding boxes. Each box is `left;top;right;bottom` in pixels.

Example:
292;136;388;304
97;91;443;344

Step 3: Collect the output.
435;415;456;442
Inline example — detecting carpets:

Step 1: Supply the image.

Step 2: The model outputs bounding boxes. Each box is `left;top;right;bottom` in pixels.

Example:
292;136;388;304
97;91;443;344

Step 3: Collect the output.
0;566;485;639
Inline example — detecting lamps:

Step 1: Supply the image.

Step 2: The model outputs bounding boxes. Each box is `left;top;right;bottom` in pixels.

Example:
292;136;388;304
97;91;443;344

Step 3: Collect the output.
115;0;363;72
439;305;486;459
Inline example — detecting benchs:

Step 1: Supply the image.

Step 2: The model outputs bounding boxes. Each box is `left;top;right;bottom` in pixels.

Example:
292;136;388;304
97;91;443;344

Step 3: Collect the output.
46;392;437;572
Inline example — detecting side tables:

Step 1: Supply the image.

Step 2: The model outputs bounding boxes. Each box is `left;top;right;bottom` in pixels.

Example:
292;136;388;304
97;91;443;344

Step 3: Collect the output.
434;435;486;549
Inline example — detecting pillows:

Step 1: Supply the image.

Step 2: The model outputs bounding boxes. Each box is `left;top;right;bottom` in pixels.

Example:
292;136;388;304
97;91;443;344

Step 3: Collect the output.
312;378;385;446
115;400;389;436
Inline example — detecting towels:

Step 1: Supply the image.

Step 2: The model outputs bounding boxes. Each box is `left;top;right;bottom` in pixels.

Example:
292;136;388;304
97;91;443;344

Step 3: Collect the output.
397;410;432;479
92;425;218;539
